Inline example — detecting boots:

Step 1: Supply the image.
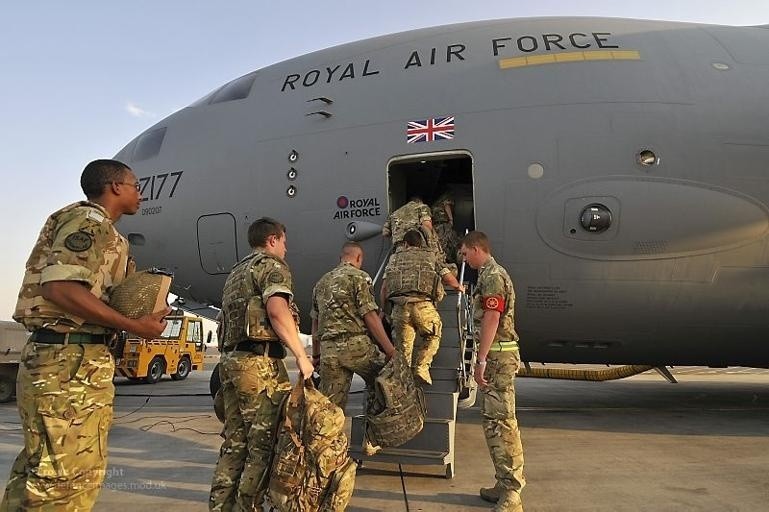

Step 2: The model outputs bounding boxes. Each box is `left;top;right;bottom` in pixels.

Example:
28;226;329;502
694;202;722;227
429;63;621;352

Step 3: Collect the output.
490;489;524;512
360;433;383;457
480;483;500;504
414;364;433;386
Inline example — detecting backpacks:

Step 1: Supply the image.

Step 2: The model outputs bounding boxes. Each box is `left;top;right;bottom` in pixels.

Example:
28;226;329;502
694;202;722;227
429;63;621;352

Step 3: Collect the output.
251;370;359;512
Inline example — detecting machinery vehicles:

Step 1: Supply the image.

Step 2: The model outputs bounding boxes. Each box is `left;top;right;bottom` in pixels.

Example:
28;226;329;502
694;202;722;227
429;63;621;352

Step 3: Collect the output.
1;315;212;403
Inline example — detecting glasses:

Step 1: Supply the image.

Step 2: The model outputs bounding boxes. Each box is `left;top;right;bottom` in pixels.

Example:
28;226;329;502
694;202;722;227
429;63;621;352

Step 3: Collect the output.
105;180;141;192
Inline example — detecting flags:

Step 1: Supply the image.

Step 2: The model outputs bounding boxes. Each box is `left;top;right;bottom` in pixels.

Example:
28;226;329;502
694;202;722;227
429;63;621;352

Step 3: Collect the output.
406;115;455;143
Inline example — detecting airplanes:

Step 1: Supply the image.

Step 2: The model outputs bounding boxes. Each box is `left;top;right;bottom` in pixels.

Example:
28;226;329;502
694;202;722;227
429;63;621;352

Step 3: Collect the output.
109;16;768;476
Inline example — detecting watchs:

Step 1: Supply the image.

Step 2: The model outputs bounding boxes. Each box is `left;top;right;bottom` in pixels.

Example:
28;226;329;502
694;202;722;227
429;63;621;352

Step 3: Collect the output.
475;358;488;367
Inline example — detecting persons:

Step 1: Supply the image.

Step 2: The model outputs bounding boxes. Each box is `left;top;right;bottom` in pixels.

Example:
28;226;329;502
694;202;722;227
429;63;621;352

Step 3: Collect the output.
208;213;316;512
381;194;446;281
457;230;526;512
1;157;174;512
379;229;465;386
307;239;397;469
431;190;456;263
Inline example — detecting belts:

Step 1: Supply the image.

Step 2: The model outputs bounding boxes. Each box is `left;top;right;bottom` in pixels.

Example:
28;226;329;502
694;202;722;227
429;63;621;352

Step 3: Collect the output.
30;331;109;344
224;341;254;351
338;332;366;339
475;340;519;352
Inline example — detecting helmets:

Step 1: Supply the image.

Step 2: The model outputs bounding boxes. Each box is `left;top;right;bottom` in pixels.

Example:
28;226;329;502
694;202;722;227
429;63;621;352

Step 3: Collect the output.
109;272;172;322
